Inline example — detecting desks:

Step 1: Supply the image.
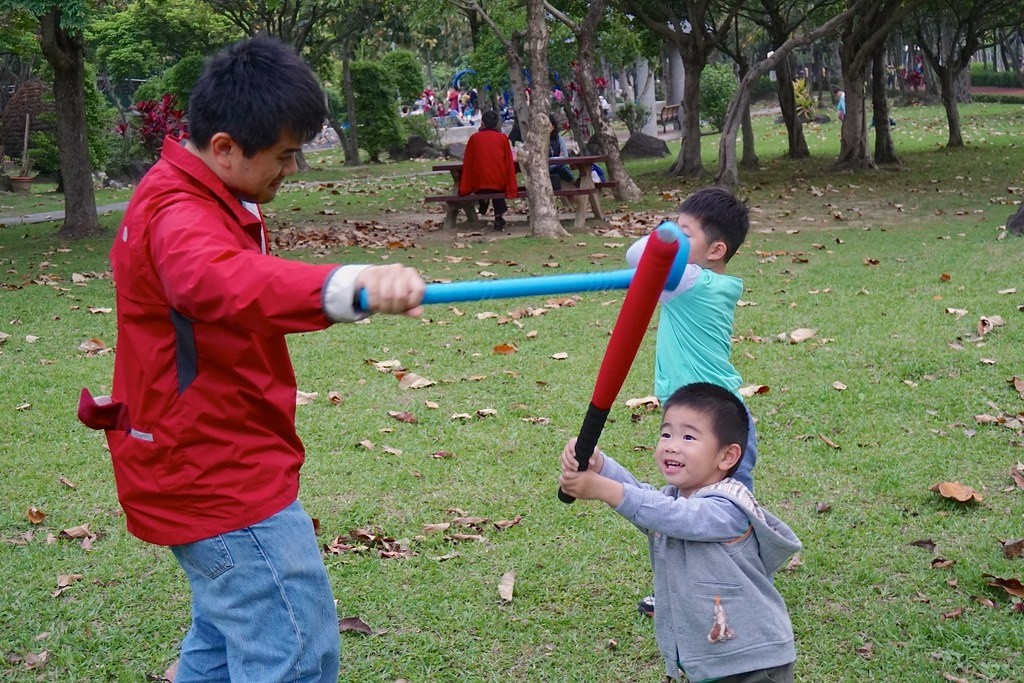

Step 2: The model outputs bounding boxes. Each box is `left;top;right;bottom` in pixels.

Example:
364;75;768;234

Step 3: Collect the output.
432;155;610;232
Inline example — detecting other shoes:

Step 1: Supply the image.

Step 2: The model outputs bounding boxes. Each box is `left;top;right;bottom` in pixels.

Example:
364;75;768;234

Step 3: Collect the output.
494;216;506;230
640;593;654;617
479;201;488;213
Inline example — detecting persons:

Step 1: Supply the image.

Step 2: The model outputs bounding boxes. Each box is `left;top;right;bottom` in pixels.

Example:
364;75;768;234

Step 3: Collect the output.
458;111;518;230
625;189;751;611
75;37;425;682
886;64;925;89
834;88;846;121
547;115;580;190
401;86;565;126
558;383;804;682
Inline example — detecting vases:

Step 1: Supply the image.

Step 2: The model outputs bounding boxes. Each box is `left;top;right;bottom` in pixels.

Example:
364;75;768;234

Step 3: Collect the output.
10;176;32;193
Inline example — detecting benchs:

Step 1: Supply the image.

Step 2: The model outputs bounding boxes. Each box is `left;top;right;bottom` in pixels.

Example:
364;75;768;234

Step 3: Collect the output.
518;180;619;197
425;186;597;211
657;104;681;134
